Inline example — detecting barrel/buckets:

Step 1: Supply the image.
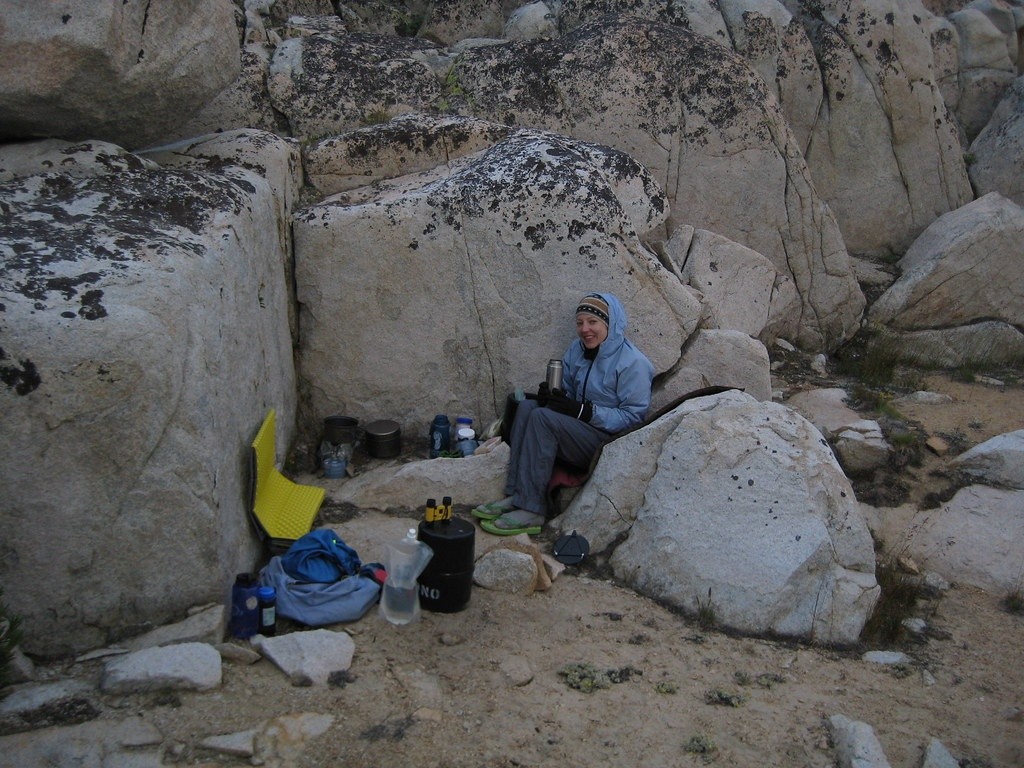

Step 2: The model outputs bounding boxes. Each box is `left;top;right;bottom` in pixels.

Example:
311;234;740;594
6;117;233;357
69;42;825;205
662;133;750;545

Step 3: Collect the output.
419;498;474;613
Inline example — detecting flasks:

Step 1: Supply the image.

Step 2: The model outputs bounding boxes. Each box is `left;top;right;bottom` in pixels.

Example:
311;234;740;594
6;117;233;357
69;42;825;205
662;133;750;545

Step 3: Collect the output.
547;358;563;392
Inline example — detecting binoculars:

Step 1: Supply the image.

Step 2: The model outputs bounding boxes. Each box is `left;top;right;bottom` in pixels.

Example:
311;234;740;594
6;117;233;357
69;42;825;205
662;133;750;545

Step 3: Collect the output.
424;496;453;526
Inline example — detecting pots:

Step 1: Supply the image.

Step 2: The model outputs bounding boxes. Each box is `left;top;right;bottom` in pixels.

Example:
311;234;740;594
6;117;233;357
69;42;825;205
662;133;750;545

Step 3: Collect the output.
324;416;358;443
365;420;401;459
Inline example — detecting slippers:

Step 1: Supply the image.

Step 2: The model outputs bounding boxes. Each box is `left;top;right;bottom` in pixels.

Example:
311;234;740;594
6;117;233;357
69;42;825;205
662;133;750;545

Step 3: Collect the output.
472;495;543;534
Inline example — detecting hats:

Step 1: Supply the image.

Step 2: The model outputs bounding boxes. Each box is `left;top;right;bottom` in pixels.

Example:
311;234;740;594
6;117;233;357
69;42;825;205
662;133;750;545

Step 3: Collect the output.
575;293;609;328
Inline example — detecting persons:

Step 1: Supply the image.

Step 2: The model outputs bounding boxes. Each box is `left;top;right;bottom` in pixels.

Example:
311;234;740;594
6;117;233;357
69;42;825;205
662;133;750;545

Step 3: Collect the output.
469;288;657;536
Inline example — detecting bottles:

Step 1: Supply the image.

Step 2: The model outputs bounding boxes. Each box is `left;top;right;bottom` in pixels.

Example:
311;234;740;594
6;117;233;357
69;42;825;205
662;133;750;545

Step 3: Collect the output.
260;588;275;638
431;415;451;458
233;574;259;638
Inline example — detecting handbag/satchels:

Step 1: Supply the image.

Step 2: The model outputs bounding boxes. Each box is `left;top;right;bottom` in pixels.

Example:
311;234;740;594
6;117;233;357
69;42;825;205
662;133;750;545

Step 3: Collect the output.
284;525;360;584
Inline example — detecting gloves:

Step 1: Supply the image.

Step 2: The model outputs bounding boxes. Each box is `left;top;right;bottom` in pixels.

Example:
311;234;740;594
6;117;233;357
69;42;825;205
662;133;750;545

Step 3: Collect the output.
537;381;549;406
547;387;592;422
